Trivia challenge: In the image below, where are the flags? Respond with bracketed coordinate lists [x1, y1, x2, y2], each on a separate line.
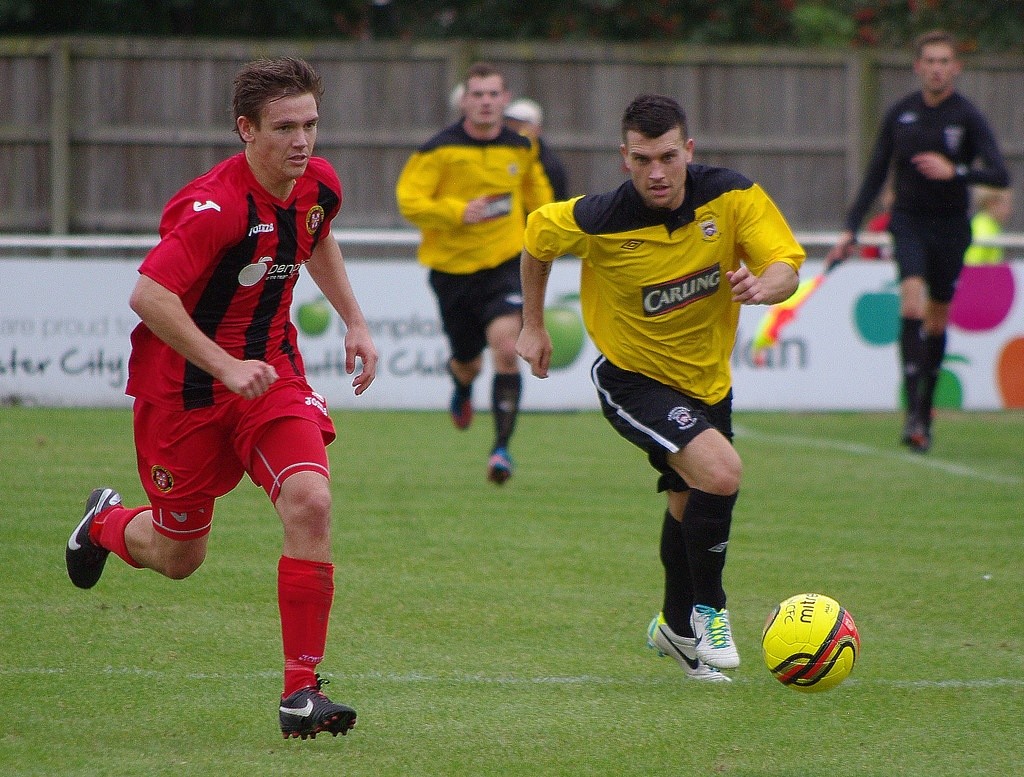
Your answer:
[753, 272, 821, 349]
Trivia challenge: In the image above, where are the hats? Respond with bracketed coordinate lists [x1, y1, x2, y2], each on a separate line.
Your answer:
[504, 99, 543, 123]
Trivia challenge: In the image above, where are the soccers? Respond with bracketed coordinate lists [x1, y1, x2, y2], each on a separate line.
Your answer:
[761, 592, 862, 692]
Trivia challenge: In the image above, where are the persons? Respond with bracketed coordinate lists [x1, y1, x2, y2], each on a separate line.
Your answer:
[63, 55, 360, 739]
[823, 32, 1012, 452]
[396, 63, 556, 486]
[861, 176, 896, 259]
[964, 184, 1012, 266]
[500, 99, 568, 219]
[515, 92, 806, 685]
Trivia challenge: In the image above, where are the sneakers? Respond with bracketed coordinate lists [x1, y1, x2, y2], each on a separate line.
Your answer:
[901, 413, 925, 443]
[647, 611, 733, 682]
[451, 385, 472, 430]
[488, 447, 512, 484]
[689, 606, 741, 670]
[915, 423, 933, 452]
[66, 488, 122, 589]
[278, 687, 357, 740]
